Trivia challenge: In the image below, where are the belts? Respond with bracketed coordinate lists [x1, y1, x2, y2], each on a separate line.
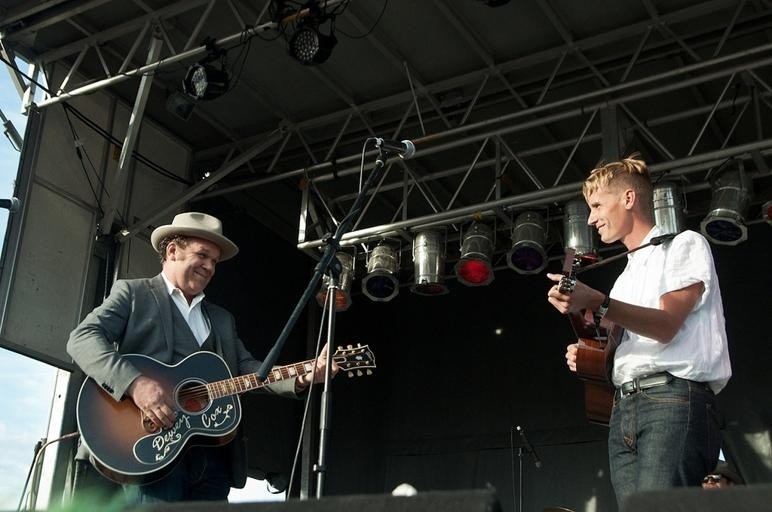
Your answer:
[613, 371, 714, 398]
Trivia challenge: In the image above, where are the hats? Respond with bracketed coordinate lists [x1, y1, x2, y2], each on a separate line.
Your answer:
[150, 212, 240, 264]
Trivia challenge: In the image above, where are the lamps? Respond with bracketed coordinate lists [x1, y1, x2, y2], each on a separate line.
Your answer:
[317, 169, 752, 312]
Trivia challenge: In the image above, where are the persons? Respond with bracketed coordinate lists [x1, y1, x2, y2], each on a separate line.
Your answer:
[701, 457, 744, 490]
[65, 209, 342, 507]
[545, 153, 734, 511]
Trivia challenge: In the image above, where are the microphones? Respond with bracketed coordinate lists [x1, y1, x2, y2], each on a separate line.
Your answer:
[61, 432, 80, 440]
[514, 426, 543, 469]
[374, 138, 416, 159]
[0, 196, 23, 212]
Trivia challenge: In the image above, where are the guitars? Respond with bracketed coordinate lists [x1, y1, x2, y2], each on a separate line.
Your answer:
[76, 342, 377, 485]
[559, 247, 624, 427]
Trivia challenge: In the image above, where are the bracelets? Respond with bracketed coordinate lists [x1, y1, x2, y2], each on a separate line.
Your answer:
[592, 296, 611, 329]
